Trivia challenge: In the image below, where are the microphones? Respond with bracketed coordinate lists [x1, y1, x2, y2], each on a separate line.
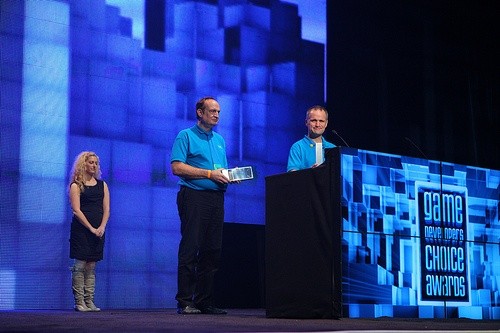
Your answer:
[405, 138, 427, 159]
[332, 130, 350, 148]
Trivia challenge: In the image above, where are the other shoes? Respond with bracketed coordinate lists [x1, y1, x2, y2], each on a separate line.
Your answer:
[202, 305, 228, 314]
[74, 302, 100, 311]
[176, 303, 201, 314]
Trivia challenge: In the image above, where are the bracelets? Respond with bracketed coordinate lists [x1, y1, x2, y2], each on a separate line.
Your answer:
[207, 170, 211, 179]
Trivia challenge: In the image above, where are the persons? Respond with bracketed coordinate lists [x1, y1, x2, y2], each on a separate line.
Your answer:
[287, 105, 337, 172]
[170, 97, 241, 315]
[69, 151, 110, 311]
